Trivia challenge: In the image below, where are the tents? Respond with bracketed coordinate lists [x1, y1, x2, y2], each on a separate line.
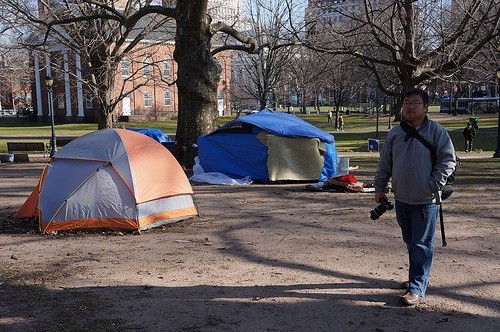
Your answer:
[197, 109, 337, 184]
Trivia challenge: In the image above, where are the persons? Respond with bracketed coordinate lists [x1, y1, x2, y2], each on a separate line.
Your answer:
[463, 122, 476, 153]
[337, 114, 344, 131]
[8, 128, 200, 235]
[327, 110, 332, 123]
[373, 88, 457, 304]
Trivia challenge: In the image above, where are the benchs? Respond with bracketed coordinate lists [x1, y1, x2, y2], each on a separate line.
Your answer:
[46, 136, 81, 151]
[7, 142, 48, 158]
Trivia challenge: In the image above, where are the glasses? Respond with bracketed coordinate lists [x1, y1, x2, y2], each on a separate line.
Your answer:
[402, 101, 422, 106]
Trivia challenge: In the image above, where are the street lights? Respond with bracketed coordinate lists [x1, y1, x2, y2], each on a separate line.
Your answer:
[43, 73, 58, 157]
[493, 68, 500, 158]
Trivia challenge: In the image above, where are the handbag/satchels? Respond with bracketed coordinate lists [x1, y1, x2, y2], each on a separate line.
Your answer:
[432, 148, 458, 185]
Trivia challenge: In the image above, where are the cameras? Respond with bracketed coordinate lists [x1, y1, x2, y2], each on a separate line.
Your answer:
[370, 197, 393, 220]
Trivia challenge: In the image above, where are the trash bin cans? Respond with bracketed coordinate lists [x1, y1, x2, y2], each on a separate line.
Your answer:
[469, 118, 479, 128]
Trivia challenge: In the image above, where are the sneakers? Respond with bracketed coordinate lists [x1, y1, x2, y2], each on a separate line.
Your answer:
[402, 281, 410, 289]
[400, 290, 421, 305]
